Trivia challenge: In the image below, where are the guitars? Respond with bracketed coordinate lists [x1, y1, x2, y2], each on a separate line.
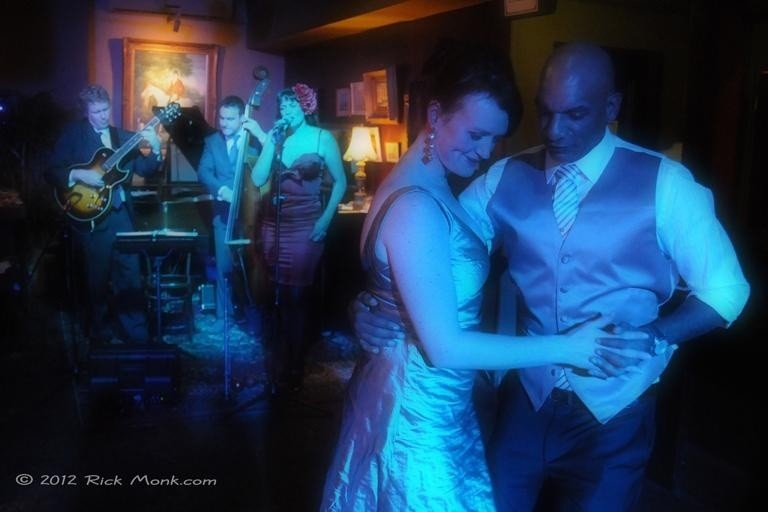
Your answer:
[54, 102, 181, 228]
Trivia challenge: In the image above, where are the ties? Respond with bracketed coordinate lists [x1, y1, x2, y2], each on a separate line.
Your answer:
[229, 135, 240, 165]
[552, 163, 581, 391]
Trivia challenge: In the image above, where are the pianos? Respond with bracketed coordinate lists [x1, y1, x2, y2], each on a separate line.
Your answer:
[124, 104, 216, 239]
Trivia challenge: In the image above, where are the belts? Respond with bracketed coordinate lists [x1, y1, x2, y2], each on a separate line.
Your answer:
[548, 389, 577, 405]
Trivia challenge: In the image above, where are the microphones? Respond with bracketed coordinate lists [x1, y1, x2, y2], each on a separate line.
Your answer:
[272, 115, 289, 136]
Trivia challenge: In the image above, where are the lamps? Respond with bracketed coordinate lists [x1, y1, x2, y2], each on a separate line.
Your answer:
[342, 125, 379, 210]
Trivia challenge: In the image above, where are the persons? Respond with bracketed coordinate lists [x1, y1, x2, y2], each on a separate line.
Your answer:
[344, 37, 750, 512]
[44, 83, 162, 345]
[316, 63, 654, 511]
[195, 95, 273, 336]
[250, 86, 347, 391]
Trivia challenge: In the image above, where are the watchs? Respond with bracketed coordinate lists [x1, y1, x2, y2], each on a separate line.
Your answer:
[642, 323, 668, 357]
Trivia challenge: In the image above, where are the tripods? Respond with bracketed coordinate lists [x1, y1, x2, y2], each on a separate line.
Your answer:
[216, 146, 334, 427]
[41, 225, 90, 421]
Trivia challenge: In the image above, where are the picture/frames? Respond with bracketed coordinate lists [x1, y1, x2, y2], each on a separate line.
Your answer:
[336, 65, 400, 126]
[503, 0, 538, 17]
[122, 34, 220, 140]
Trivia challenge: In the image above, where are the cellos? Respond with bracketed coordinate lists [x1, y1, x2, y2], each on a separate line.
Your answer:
[229, 69, 276, 313]
